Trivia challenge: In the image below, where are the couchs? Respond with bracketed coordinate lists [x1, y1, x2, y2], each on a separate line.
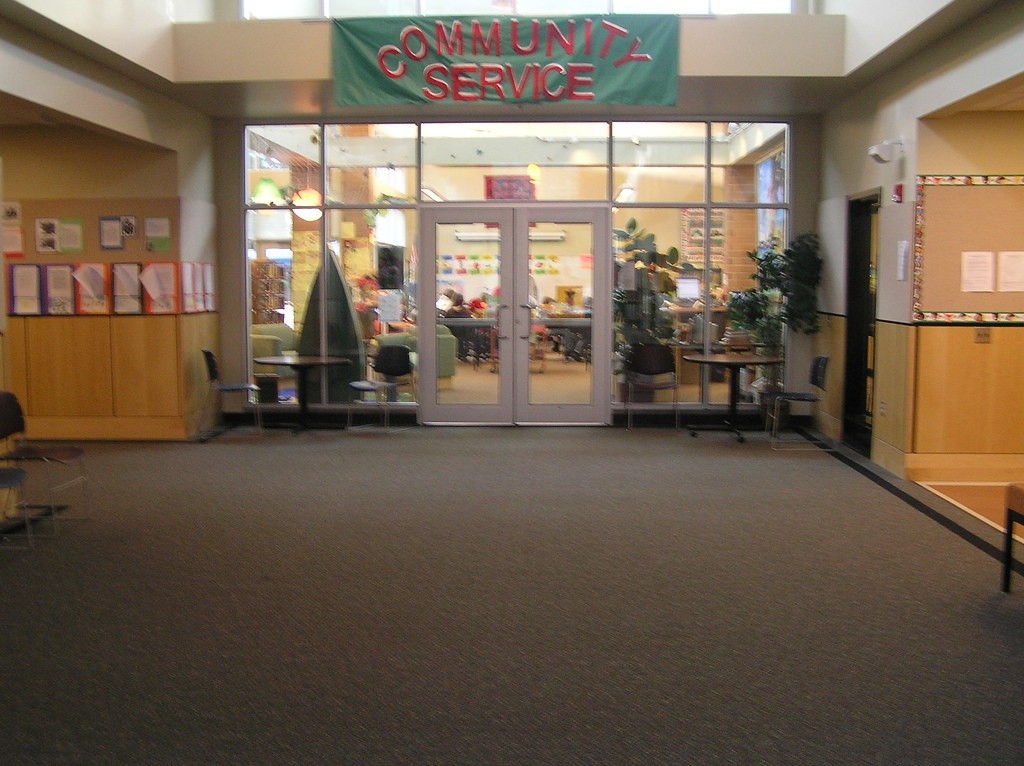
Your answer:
[247, 322, 457, 379]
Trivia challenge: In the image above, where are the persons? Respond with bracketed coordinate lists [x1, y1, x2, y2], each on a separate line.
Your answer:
[436, 289, 499, 355]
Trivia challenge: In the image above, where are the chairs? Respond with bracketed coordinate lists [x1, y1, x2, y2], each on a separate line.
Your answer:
[347, 344, 424, 435]
[626, 344, 680, 433]
[0, 391, 94, 551]
[762, 355, 840, 452]
[193, 346, 267, 444]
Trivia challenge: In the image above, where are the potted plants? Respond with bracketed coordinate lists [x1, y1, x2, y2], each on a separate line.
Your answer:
[609, 216, 696, 404]
[727, 229, 825, 407]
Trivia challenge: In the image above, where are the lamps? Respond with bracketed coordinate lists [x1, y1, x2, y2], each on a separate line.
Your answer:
[612, 185, 638, 204]
[249, 176, 325, 221]
[455, 230, 568, 242]
[370, 161, 407, 248]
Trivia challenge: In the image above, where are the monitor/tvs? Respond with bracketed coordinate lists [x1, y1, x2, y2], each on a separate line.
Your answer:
[675, 276, 701, 302]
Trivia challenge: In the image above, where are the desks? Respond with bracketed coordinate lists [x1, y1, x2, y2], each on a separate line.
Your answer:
[252, 356, 353, 435]
[682, 351, 785, 445]
